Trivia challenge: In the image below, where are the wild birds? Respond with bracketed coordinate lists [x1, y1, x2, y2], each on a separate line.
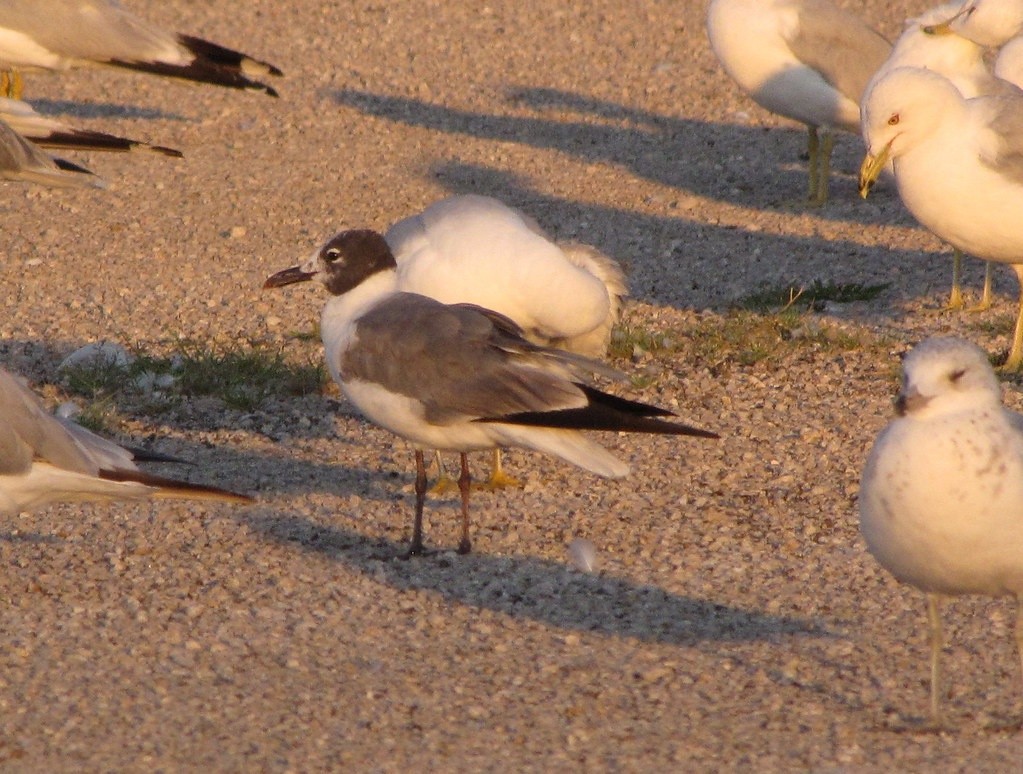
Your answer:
[0, 345, 257, 540]
[0, 1, 284, 191]
[859, 0, 1023, 379]
[259, 188, 722, 568]
[858, 336, 1023, 736]
[706, 1, 896, 211]
